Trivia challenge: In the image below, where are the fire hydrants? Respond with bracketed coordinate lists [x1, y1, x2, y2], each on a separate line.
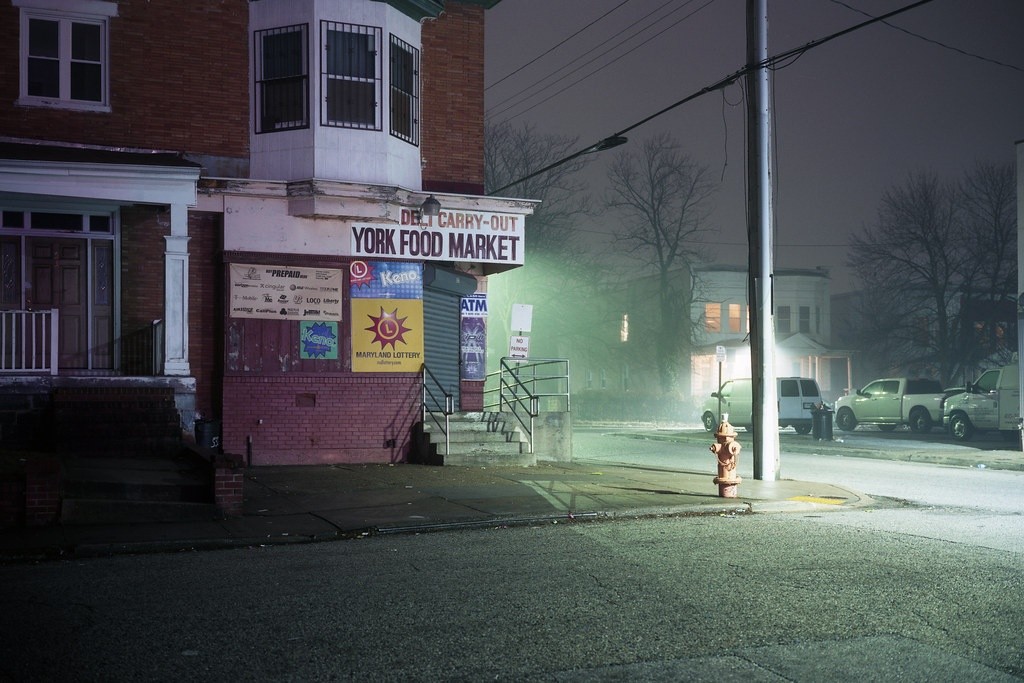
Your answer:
[707, 413, 743, 497]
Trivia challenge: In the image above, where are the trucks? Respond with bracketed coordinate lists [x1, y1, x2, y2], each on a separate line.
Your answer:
[944, 364, 1023, 441]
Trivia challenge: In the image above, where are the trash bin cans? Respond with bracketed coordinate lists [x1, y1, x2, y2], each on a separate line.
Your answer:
[810, 401, 835, 441]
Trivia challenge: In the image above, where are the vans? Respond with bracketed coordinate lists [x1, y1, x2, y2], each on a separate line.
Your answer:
[701, 376, 823, 435]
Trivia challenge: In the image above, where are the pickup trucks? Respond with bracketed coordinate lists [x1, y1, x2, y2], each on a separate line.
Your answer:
[833, 378, 959, 432]
